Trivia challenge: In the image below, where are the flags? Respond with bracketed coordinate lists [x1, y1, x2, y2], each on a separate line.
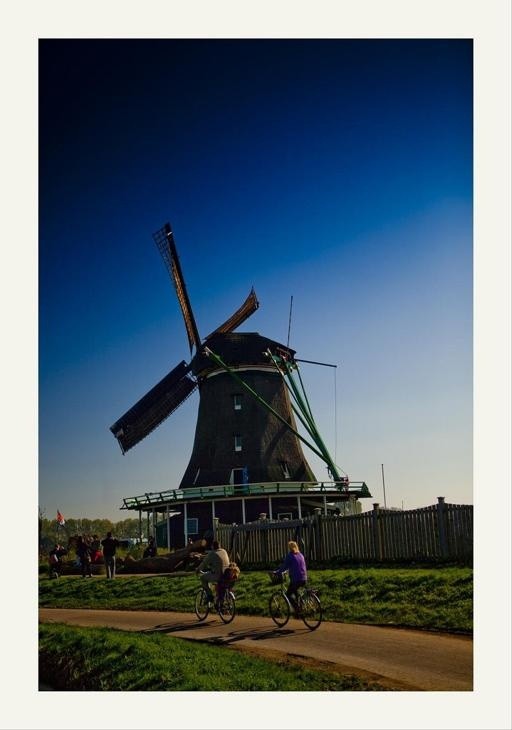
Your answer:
[57, 511, 65, 525]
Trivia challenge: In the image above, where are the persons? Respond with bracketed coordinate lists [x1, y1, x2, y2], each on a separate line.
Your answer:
[143, 536, 157, 559]
[195, 541, 229, 602]
[76, 532, 118, 579]
[214, 563, 240, 601]
[273, 541, 307, 619]
[49, 545, 68, 579]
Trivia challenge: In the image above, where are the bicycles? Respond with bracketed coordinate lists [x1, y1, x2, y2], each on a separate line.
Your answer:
[266, 570, 324, 631]
[194, 568, 237, 624]
